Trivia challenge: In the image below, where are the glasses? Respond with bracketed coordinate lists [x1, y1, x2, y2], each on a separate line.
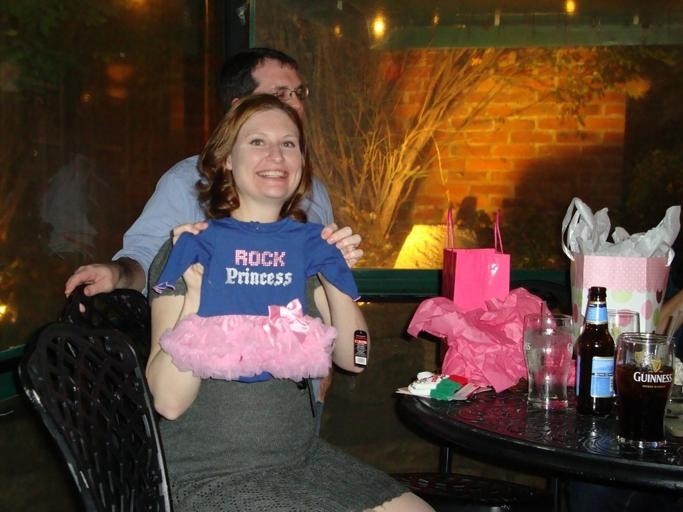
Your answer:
[268, 82, 311, 102]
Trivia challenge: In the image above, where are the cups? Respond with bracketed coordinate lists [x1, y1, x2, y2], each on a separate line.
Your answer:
[614, 332, 675, 447]
[522, 313, 574, 408]
[574, 287, 617, 419]
[605, 308, 640, 347]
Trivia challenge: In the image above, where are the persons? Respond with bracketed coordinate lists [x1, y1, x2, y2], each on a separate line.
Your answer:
[63, 46, 366, 315]
[141, 90, 439, 512]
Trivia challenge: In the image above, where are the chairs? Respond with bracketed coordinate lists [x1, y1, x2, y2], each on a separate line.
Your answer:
[59, 284, 161, 426]
[14, 323, 170, 511]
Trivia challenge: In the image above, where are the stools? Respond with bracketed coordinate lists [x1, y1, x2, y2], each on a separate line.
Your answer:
[387, 472, 549, 512]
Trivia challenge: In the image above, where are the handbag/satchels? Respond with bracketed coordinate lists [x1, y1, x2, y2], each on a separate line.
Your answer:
[441, 247, 512, 390]
[569, 249, 676, 359]
